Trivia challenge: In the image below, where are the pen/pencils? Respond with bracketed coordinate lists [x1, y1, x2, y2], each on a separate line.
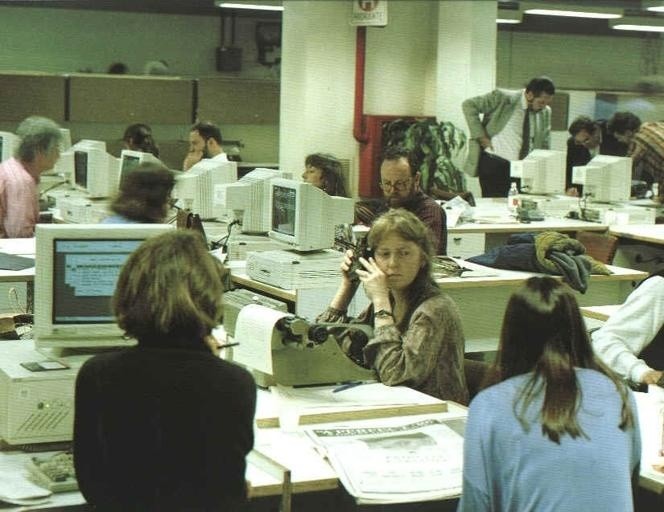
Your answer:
[332, 381, 364, 395]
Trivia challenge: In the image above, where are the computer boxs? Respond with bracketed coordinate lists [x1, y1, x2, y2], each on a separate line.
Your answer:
[507, 195, 578, 218]
[577, 202, 657, 229]
[44, 190, 111, 225]
[247, 249, 348, 291]
[0, 339, 100, 446]
[206, 229, 271, 261]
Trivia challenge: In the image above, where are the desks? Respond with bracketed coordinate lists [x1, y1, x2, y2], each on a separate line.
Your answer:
[576, 303, 628, 322]
[350, 197, 609, 258]
[628, 384, 664, 492]
[0, 325, 469, 512]
[518, 193, 663, 245]
[229, 253, 649, 341]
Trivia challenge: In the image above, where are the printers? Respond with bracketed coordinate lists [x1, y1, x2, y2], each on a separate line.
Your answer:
[222, 287, 383, 389]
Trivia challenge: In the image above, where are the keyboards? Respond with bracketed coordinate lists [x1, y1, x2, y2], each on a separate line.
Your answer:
[26, 453, 79, 494]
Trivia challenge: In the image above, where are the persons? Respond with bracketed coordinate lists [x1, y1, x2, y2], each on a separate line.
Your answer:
[101, 162, 178, 224]
[184, 121, 228, 173]
[590, 268, 664, 392]
[607, 112, 664, 193]
[356, 149, 447, 256]
[315, 208, 470, 407]
[301, 153, 346, 197]
[566, 115, 627, 199]
[0, 115, 62, 238]
[124, 123, 159, 158]
[73, 230, 257, 512]
[457, 276, 641, 512]
[462, 76, 556, 198]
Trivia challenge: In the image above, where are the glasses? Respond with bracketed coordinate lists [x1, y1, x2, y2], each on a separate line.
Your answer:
[379, 178, 410, 192]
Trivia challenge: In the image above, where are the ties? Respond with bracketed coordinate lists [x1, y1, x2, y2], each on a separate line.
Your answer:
[518, 110, 530, 160]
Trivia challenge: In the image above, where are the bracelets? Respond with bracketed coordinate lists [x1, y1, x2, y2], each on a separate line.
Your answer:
[374, 309, 393, 318]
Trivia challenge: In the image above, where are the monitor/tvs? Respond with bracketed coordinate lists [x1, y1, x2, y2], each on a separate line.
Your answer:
[0, 131, 21, 162]
[212, 166, 294, 236]
[266, 176, 356, 253]
[572, 155, 634, 204]
[507, 147, 567, 196]
[33, 223, 176, 348]
[54, 138, 107, 179]
[70, 145, 118, 199]
[172, 157, 237, 222]
[113, 149, 165, 198]
